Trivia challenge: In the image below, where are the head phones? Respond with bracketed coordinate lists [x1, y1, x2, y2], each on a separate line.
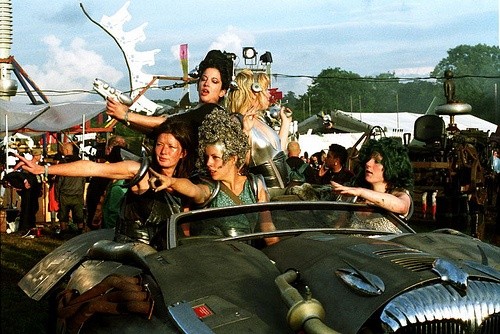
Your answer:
[251, 73, 262, 92]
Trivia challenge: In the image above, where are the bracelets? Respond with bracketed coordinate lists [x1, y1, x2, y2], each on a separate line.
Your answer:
[44, 165, 48, 176]
[124, 111, 129, 122]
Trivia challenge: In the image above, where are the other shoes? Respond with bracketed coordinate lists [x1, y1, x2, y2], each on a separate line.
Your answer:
[22, 230, 34, 239]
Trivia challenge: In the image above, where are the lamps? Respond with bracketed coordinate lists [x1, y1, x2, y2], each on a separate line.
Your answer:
[243, 47, 272, 68]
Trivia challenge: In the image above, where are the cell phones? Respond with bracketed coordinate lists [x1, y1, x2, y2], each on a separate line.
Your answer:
[325, 164, 330, 169]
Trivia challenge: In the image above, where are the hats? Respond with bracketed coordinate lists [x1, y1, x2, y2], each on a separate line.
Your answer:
[32, 149, 42, 156]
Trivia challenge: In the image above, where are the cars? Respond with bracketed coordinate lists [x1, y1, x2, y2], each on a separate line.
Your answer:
[17, 198, 500, 334]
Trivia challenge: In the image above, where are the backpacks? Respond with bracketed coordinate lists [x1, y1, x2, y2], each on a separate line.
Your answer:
[285, 161, 309, 184]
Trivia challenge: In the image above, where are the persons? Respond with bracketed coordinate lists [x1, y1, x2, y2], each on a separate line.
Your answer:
[105, 49, 234, 173]
[148, 108, 279, 245]
[227, 70, 293, 189]
[0, 132, 127, 236]
[286, 122, 500, 237]
[13, 120, 190, 251]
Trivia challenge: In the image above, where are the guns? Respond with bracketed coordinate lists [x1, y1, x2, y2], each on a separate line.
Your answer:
[270, 104, 293, 119]
[92, 77, 134, 107]
